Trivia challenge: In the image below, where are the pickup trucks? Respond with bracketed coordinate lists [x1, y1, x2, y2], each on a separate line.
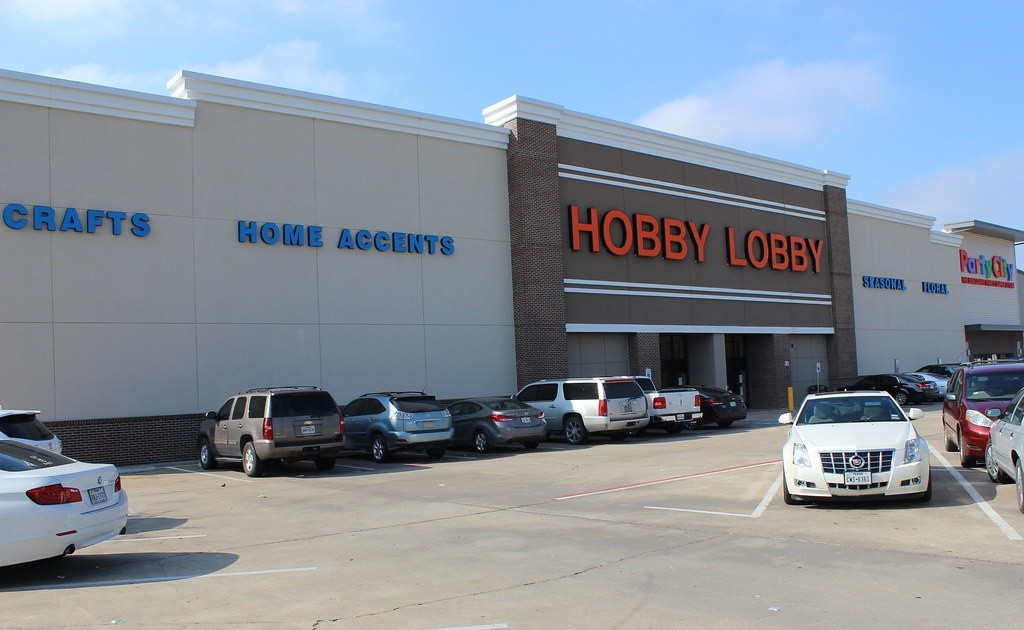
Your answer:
[594, 375, 704, 435]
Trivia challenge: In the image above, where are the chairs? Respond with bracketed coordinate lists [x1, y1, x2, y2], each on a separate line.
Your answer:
[808, 401, 836, 423]
[970, 378, 995, 394]
[861, 405, 889, 419]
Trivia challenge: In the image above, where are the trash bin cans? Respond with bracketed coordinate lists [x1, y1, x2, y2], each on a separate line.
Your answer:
[808, 384, 830, 395]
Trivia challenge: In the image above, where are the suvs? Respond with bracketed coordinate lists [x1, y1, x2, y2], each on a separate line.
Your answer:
[334, 391, 456, 464]
[942, 358, 1024, 468]
[197, 385, 347, 477]
[510, 376, 650, 446]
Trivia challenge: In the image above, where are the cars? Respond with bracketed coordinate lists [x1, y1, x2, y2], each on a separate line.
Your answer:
[836, 373, 940, 405]
[778, 390, 932, 504]
[0, 408, 64, 459]
[661, 384, 747, 430]
[913, 362, 979, 388]
[0, 439, 130, 570]
[901, 371, 952, 402]
[443, 396, 549, 453]
[984, 387, 1024, 513]
